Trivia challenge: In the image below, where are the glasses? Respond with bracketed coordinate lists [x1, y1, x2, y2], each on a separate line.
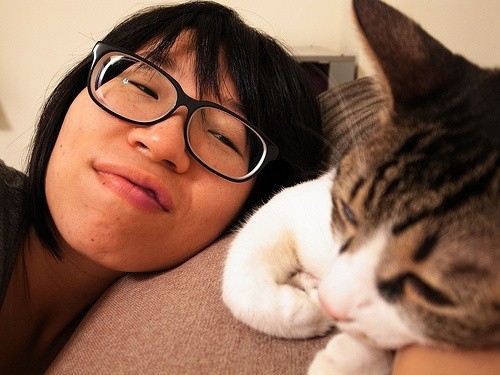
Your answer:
[87, 40, 278, 182]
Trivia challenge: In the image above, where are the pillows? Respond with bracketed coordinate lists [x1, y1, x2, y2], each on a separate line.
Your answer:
[312, 76, 388, 169]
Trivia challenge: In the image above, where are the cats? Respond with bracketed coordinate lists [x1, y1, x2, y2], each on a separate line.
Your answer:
[219, 0, 500, 375]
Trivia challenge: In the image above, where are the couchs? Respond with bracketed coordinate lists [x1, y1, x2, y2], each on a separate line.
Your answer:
[42, 212, 500, 375]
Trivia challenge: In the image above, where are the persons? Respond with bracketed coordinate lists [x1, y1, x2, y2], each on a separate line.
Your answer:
[1, 1, 326, 375]
